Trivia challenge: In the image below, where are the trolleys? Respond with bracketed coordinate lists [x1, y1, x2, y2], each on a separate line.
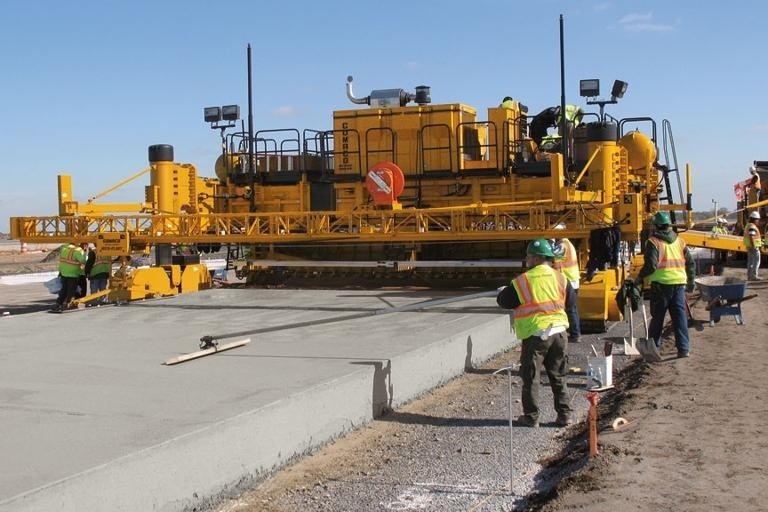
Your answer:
[694, 275, 758, 327]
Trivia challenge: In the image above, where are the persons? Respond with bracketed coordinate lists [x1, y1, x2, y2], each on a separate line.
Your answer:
[495, 240, 573, 429]
[722, 217, 730, 268]
[710, 218, 724, 270]
[77, 242, 95, 299]
[742, 211, 764, 282]
[498, 95, 528, 115]
[635, 211, 697, 356]
[51, 242, 89, 313]
[88, 246, 113, 305]
[551, 223, 582, 343]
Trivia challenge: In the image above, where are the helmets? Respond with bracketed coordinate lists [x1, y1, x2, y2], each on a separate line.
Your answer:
[749, 211, 761, 220]
[652, 211, 671, 226]
[717, 218, 727, 224]
[527, 239, 555, 258]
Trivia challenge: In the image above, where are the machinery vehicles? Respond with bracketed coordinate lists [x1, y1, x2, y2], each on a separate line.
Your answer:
[10, 13, 768, 332]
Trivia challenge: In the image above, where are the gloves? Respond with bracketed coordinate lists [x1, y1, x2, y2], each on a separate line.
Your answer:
[633, 276, 644, 286]
[684, 285, 695, 292]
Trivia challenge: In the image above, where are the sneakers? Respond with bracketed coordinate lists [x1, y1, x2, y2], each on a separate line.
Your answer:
[567, 335, 582, 343]
[556, 416, 572, 426]
[518, 414, 540, 428]
[749, 276, 763, 281]
[678, 351, 690, 358]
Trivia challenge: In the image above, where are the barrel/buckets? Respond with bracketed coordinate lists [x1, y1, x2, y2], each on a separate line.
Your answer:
[585, 351, 613, 387]
[44, 275, 62, 294]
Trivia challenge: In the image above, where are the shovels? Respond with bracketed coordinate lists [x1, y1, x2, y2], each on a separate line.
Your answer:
[624, 297, 662, 362]
